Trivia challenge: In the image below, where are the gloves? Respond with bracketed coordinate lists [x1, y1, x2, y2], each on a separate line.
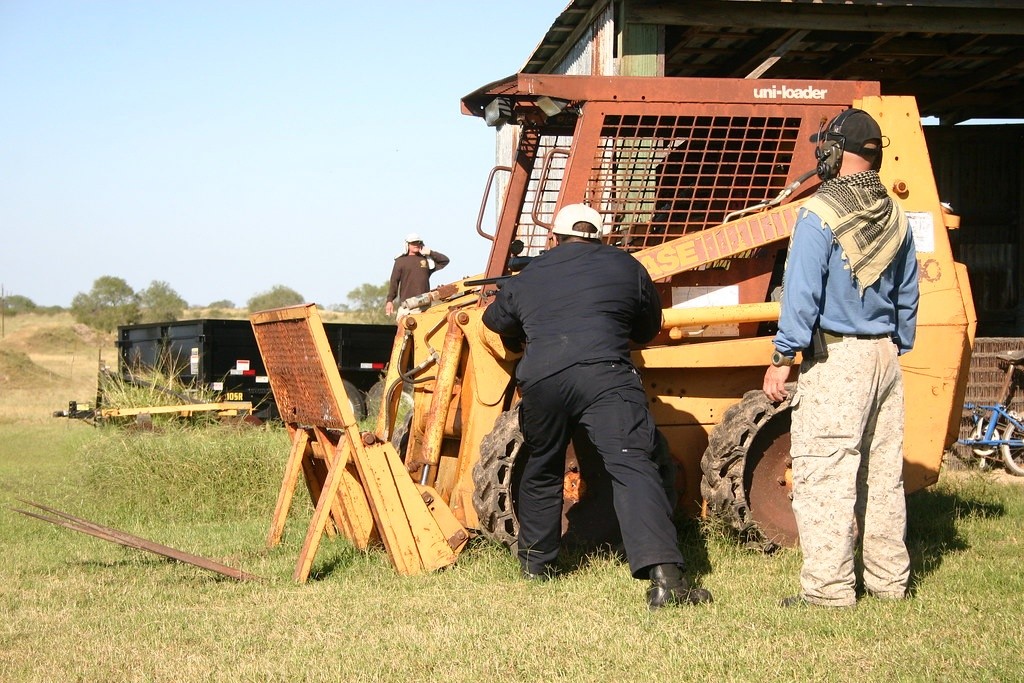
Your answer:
[419, 246, 431, 255]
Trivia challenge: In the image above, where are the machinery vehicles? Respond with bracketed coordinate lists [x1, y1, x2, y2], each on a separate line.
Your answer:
[247, 72, 975, 566]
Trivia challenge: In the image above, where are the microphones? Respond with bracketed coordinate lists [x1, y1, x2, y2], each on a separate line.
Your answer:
[815, 115, 828, 159]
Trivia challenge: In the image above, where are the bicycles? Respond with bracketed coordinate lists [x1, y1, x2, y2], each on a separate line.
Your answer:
[956, 350, 1024, 477]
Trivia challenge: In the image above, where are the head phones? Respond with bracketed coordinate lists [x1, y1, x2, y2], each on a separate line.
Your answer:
[814, 108, 884, 180]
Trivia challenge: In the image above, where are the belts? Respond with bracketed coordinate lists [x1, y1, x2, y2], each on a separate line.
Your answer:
[822, 330, 888, 345]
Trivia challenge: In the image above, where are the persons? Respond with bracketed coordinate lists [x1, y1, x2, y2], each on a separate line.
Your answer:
[385, 232, 451, 321]
[762, 107, 922, 612]
[481, 203, 715, 609]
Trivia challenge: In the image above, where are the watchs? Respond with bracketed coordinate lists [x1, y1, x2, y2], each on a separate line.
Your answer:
[770, 351, 792, 367]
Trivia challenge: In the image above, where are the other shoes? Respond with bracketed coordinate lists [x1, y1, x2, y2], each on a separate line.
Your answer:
[781, 594, 808, 607]
[647, 576, 715, 612]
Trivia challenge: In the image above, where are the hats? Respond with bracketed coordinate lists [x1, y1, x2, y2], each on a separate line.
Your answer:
[394, 233, 436, 270]
[808, 107, 882, 157]
[552, 202, 604, 240]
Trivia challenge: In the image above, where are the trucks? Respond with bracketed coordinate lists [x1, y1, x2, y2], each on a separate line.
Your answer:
[113, 318, 414, 422]
